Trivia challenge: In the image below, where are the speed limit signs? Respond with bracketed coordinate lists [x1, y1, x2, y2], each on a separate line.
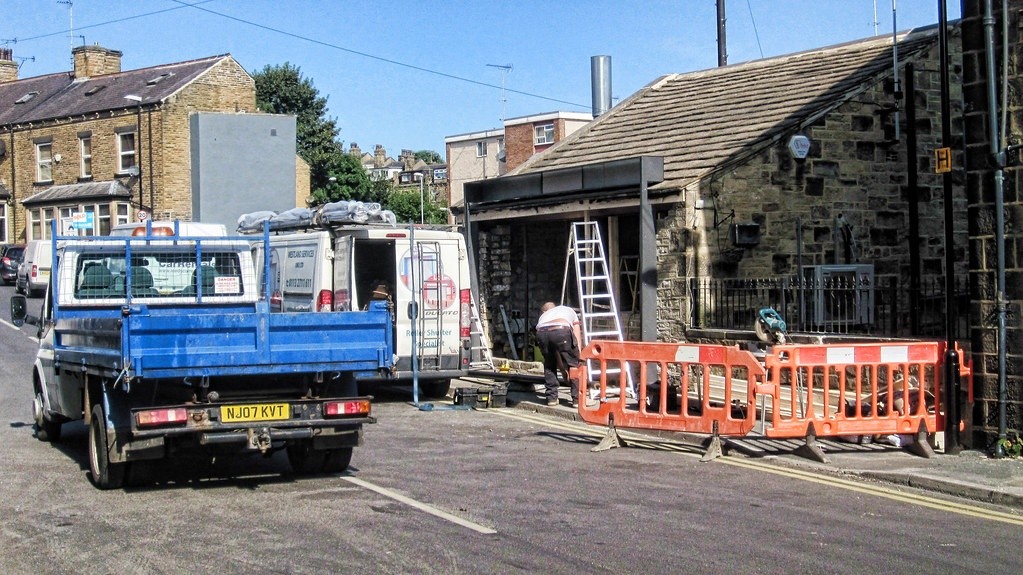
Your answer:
[137, 210, 147, 219]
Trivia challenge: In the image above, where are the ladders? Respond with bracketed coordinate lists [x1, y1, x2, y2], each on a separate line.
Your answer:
[560, 220, 637, 402]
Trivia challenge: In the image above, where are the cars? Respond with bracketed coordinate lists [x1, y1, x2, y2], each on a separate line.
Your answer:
[0, 228, 62, 291]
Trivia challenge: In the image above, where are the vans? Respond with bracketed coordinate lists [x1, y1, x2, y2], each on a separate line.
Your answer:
[234, 201, 480, 401]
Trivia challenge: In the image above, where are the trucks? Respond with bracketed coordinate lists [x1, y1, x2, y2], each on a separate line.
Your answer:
[30, 219, 397, 487]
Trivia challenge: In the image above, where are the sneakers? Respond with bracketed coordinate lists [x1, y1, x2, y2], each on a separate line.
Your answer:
[572, 398, 596, 407]
[548, 399, 559, 406]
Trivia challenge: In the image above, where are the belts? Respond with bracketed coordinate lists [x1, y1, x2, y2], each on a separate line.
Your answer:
[537, 326, 569, 331]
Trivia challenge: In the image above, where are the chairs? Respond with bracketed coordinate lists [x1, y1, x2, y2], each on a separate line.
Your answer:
[73, 265, 116, 299]
[116, 267, 161, 297]
[184, 265, 219, 294]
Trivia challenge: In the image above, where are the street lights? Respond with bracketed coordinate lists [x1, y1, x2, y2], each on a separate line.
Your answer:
[414, 171, 424, 224]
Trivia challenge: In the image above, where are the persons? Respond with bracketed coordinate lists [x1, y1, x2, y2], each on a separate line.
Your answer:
[536, 301, 585, 409]
[82, 261, 101, 278]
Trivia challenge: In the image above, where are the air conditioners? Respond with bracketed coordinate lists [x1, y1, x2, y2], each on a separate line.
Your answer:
[802, 264, 875, 327]
